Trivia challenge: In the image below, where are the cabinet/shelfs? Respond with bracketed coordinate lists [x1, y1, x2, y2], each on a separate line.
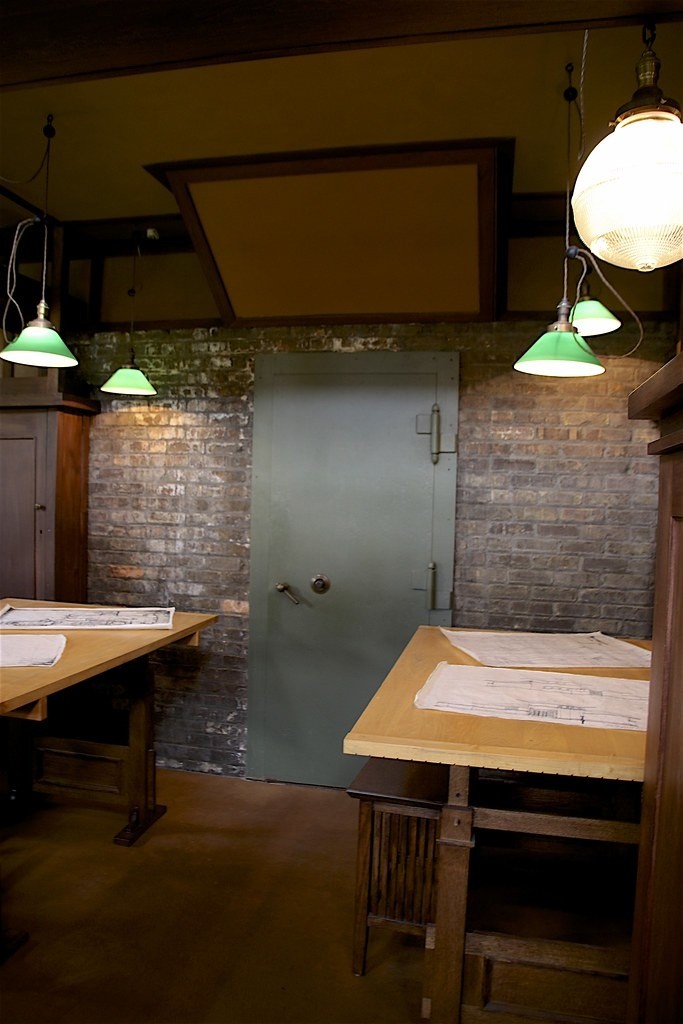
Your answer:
[0, 396, 102, 603]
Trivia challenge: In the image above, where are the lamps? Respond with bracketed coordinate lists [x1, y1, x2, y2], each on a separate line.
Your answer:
[0, 115, 82, 367]
[515, 62, 609, 376]
[561, 21, 683, 274]
[569, 26, 624, 337]
[101, 244, 159, 397]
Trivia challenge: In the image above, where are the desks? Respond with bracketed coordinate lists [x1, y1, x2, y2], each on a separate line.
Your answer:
[0, 599, 224, 848]
[351, 625, 655, 1024]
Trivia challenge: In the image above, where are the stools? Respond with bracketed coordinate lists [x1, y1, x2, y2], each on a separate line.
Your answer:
[351, 754, 480, 973]
